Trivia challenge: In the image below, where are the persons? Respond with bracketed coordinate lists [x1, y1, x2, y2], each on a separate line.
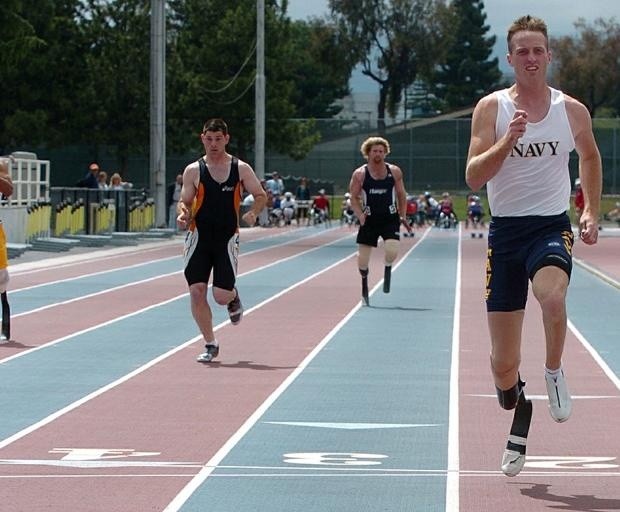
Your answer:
[348, 136, 413, 308]
[76, 163, 133, 232]
[393, 189, 458, 237]
[466, 188, 491, 239]
[0, 157, 19, 345]
[177, 115, 271, 364]
[573, 177, 602, 237]
[166, 174, 183, 225]
[342, 188, 364, 226]
[465, 13, 605, 477]
[603, 199, 620, 223]
[239, 171, 331, 228]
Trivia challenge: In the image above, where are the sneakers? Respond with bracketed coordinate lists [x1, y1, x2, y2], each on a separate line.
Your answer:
[227, 287, 243, 325]
[546, 369, 572, 423]
[196, 343, 219, 362]
